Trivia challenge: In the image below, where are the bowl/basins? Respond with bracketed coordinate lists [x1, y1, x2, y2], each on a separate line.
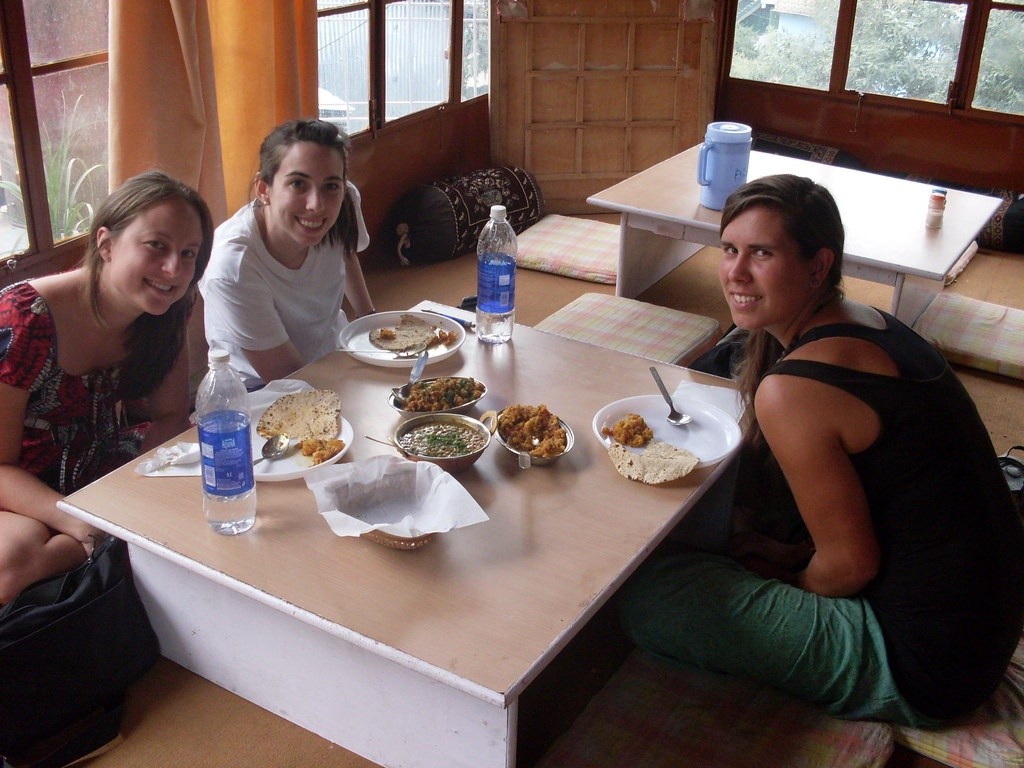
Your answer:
[388, 376, 488, 420]
[491, 408, 576, 466]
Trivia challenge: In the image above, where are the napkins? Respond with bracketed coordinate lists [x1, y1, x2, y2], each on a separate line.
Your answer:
[134, 441, 201, 477]
[247, 379, 315, 409]
[671, 380, 752, 424]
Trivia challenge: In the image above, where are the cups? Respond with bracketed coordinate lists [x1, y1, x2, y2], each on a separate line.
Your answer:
[698, 121, 753, 211]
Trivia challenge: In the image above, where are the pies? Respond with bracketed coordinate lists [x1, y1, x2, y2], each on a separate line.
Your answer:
[256, 389, 342, 441]
[607, 441, 700, 485]
[369, 314, 436, 350]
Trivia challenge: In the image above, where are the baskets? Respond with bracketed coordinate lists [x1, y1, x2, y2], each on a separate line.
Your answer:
[327, 466, 439, 551]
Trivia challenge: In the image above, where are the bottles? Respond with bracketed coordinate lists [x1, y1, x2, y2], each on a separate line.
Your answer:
[195, 349, 256, 536]
[476, 206, 517, 343]
[926, 187, 947, 232]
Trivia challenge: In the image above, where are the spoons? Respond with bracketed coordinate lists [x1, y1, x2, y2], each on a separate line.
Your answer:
[253, 433, 290, 470]
[334, 343, 428, 357]
[649, 366, 692, 426]
[391, 351, 428, 404]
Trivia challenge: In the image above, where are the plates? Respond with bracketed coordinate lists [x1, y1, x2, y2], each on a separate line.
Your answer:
[247, 409, 354, 482]
[339, 311, 466, 369]
[593, 395, 743, 470]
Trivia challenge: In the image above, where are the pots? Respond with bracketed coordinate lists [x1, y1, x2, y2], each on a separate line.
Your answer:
[388, 411, 499, 476]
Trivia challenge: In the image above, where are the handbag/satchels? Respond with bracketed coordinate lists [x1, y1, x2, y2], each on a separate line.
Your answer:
[0, 535, 162, 768]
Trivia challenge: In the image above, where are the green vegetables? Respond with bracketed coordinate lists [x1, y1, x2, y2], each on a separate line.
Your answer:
[425, 432, 468, 455]
[408, 376, 477, 408]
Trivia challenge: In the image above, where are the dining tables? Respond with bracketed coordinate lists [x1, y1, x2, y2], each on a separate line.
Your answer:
[54, 298, 757, 768]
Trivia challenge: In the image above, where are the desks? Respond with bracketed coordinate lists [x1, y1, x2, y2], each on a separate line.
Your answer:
[586, 142, 1003, 330]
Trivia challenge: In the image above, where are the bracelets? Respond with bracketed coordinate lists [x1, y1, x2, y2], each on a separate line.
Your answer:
[355, 309, 376, 319]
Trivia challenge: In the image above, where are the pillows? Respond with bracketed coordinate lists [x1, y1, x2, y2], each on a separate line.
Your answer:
[752, 131, 864, 172]
[396, 164, 543, 267]
[860, 168, 1024, 256]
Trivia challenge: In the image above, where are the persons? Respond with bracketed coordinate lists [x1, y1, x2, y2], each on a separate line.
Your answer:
[196, 120, 374, 392]
[614, 174, 1024, 732]
[0, 171, 212, 606]
